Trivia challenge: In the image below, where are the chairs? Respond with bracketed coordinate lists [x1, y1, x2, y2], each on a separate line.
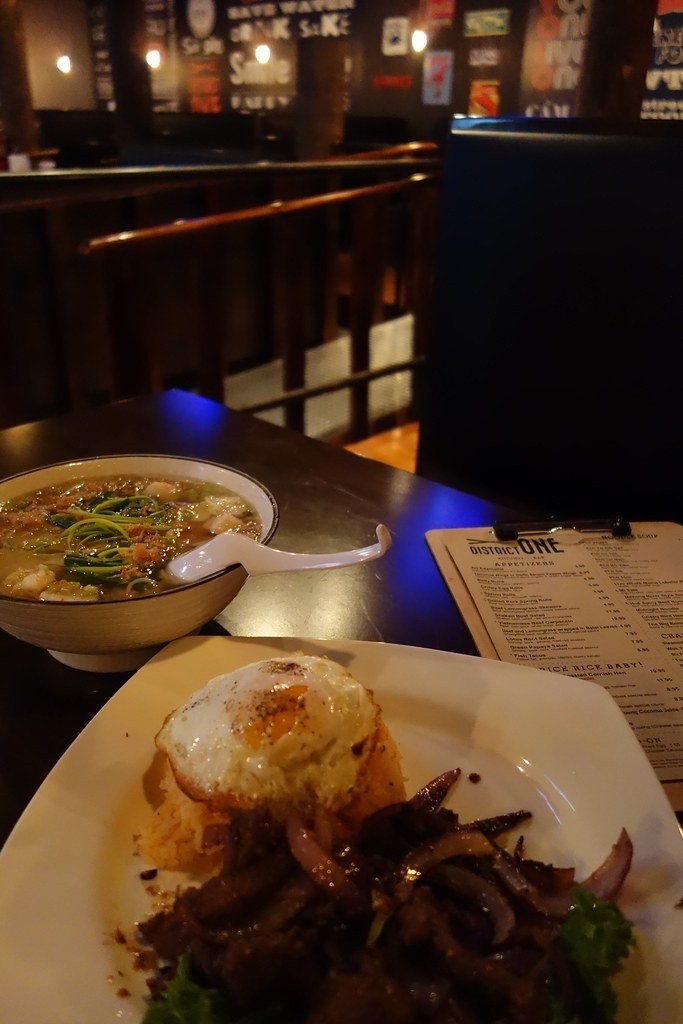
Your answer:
[414, 117, 683, 524]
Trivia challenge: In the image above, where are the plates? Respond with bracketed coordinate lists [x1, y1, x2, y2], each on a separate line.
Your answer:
[0, 635, 683, 1024]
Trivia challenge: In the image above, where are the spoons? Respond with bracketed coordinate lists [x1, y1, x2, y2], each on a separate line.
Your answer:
[167, 524, 399, 583]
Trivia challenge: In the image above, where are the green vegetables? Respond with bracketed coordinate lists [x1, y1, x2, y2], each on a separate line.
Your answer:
[142, 882, 638, 1024]
[24, 489, 192, 594]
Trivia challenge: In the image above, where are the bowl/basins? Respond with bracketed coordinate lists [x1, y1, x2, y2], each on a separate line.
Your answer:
[1, 454, 280, 673]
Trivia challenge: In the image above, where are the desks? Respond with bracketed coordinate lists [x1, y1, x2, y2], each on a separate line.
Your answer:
[0, 387, 683, 850]
